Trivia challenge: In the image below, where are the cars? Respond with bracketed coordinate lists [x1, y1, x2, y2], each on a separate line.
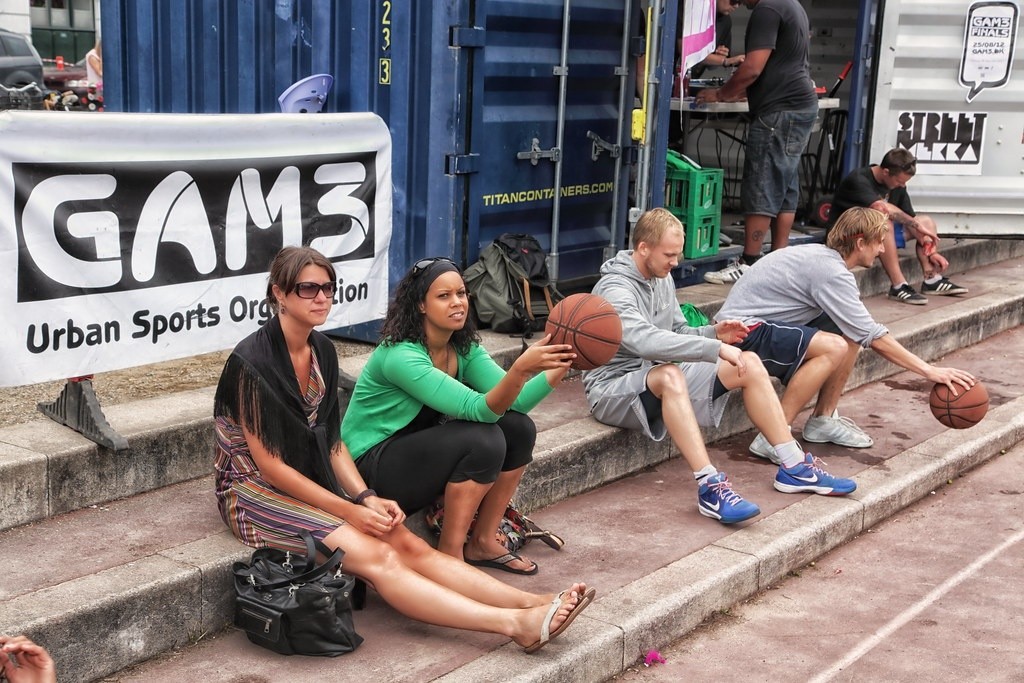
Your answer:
[44, 56, 88, 105]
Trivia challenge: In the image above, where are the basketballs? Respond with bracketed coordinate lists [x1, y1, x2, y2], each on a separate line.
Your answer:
[929, 376, 990, 430]
[545, 292, 623, 370]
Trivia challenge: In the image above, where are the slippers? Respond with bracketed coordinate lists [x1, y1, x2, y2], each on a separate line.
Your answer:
[525, 586, 596, 652]
[466, 547, 538, 575]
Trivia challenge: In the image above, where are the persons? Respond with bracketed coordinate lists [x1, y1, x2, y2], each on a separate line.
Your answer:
[581, 208, 856, 523]
[213, 246, 595, 655]
[340, 260, 577, 575]
[87, 43, 102, 85]
[714, 208, 974, 465]
[669, 0, 746, 151]
[0, 635, 56, 683]
[826, 149, 969, 305]
[697, 0, 818, 285]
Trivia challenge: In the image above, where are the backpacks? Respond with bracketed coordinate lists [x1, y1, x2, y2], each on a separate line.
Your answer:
[462, 232, 565, 337]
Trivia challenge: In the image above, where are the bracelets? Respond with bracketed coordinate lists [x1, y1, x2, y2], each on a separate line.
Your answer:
[723, 58, 727, 68]
[354, 489, 377, 505]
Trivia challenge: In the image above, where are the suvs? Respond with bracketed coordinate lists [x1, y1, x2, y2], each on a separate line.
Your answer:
[0, 28, 44, 110]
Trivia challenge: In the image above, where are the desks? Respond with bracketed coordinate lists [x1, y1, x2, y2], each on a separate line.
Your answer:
[671, 96, 840, 227]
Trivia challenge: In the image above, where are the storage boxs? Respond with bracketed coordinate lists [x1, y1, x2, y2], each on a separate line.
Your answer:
[664, 166, 724, 260]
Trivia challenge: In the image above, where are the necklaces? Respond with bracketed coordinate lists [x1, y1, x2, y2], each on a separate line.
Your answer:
[447, 344, 448, 374]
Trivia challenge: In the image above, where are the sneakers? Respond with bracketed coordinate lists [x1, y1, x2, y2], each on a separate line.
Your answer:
[774, 452, 857, 495]
[889, 284, 928, 304]
[922, 277, 968, 295]
[697, 472, 760, 523]
[749, 426, 805, 466]
[802, 408, 874, 447]
[703, 252, 764, 284]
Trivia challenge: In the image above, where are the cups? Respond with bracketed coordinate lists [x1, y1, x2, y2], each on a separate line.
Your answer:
[56, 56, 64, 70]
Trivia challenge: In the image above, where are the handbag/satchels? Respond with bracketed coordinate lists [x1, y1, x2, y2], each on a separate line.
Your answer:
[425, 499, 565, 553]
[231, 530, 364, 657]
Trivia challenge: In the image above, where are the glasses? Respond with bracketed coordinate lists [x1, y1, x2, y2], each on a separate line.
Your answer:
[729, 0, 742, 6]
[285, 281, 336, 299]
[411, 257, 456, 280]
